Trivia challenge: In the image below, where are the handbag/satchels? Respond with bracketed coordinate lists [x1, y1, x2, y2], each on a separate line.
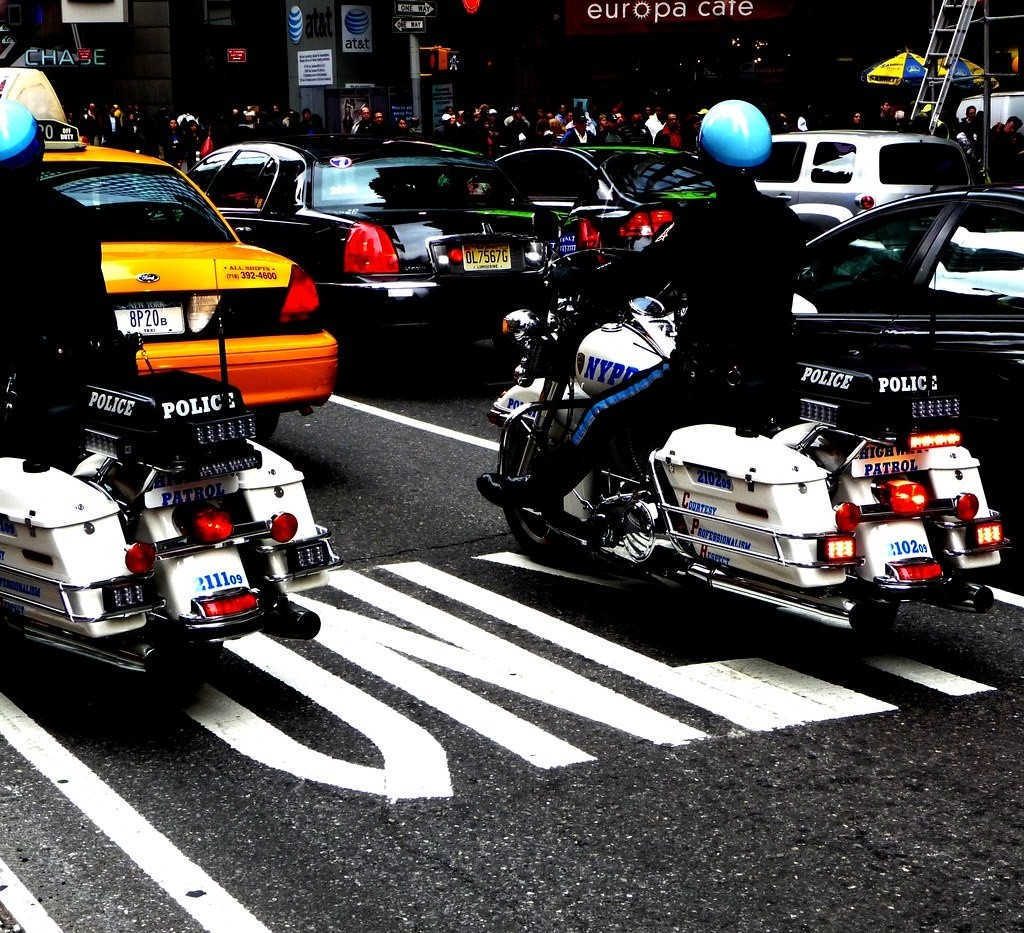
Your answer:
[200, 127, 213, 158]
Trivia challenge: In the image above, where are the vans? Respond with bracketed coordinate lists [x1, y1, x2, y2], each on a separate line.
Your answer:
[748, 129, 980, 241]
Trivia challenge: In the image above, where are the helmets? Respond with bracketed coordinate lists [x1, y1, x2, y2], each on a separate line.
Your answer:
[700, 100, 773, 177]
[0, 84, 44, 171]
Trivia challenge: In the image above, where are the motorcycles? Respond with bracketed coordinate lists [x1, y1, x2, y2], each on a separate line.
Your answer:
[483, 224, 1005, 636]
[0, 341, 344, 711]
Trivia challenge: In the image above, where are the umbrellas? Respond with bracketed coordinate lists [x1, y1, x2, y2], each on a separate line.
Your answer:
[860, 51, 951, 86]
[934, 57, 999, 89]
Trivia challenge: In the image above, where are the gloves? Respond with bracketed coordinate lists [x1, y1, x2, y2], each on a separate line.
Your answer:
[547, 264, 585, 294]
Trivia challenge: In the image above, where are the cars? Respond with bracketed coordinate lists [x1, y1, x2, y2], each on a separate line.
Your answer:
[804, 184, 1024, 512]
[175, 131, 580, 359]
[0, 65, 342, 453]
[462, 145, 727, 249]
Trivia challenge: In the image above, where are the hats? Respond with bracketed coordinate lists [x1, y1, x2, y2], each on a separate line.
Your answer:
[506, 103, 700, 131]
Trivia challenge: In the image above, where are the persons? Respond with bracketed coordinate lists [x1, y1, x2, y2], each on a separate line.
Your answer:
[478, 99, 805, 507]
[0, 105, 122, 461]
[65, 103, 1024, 186]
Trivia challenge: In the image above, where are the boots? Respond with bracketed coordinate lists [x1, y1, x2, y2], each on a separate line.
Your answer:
[479, 437, 593, 521]
